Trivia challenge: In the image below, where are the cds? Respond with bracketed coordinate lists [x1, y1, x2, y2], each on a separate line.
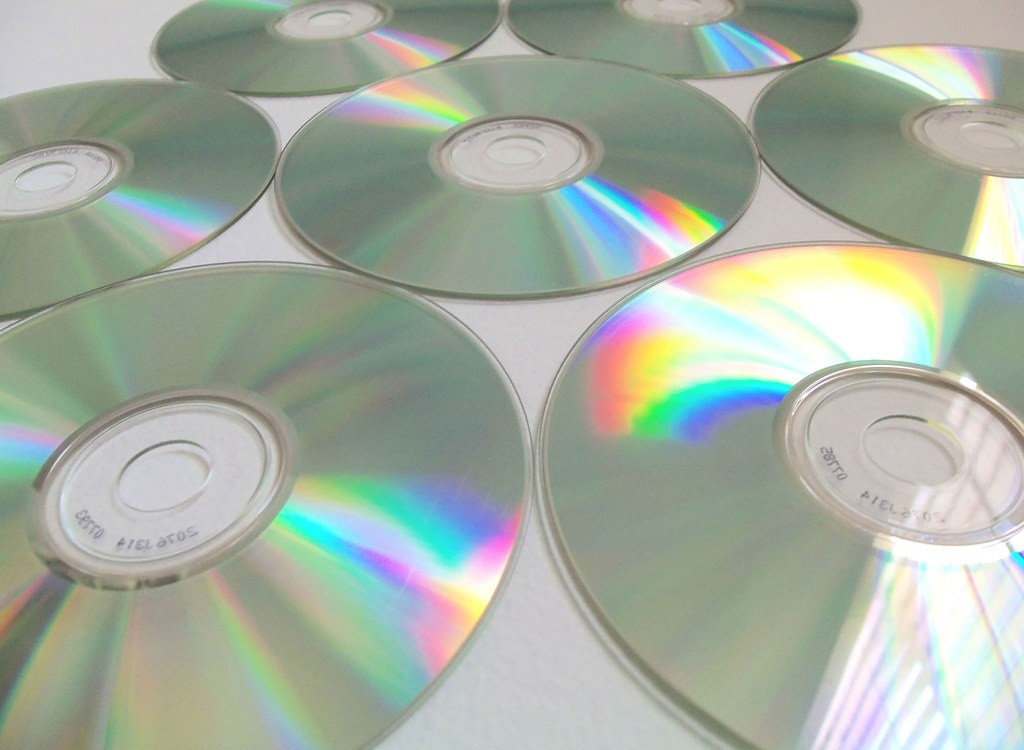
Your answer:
[1, 0, 1023, 750]
